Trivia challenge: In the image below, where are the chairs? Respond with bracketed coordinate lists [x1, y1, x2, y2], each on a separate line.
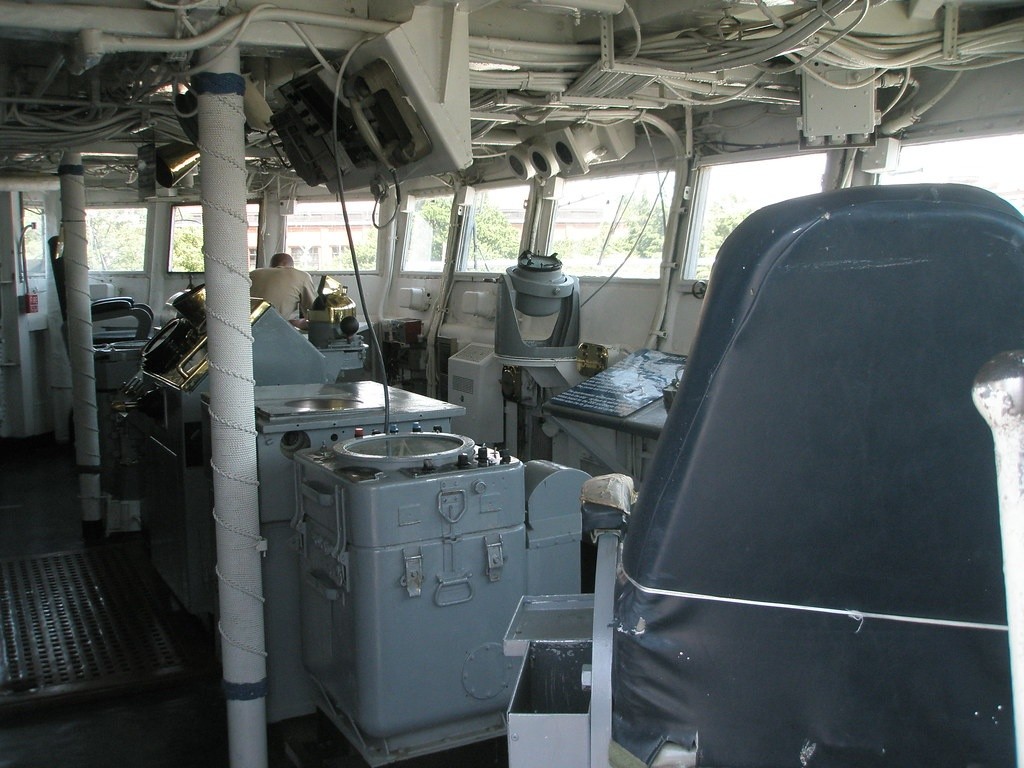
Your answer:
[578, 184, 1024, 768]
[48, 234, 154, 359]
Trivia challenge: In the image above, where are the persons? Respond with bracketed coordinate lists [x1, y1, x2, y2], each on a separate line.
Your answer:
[250, 252, 317, 330]
